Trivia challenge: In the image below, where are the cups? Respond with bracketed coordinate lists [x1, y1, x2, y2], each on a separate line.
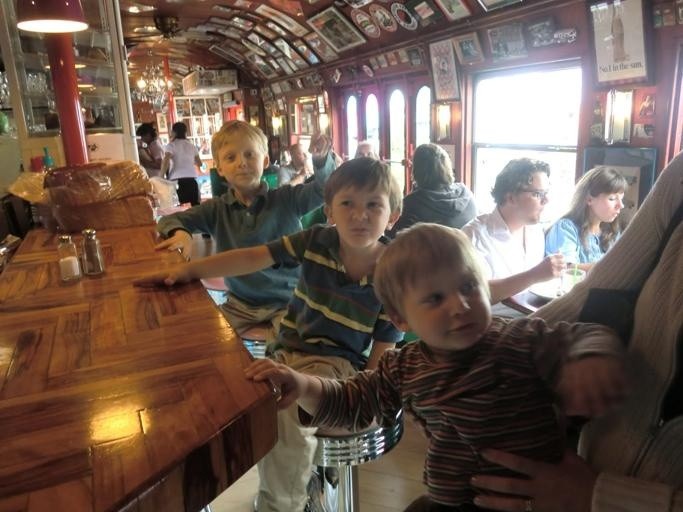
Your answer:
[562, 268, 587, 293]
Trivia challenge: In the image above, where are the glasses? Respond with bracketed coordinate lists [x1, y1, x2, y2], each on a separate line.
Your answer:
[523, 189, 549, 200]
[519, 188, 550, 200]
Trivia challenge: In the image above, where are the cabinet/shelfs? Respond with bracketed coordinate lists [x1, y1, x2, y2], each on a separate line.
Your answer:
[0, 0, 140, 172]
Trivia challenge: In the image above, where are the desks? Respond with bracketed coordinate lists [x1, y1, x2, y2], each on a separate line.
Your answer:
[499, 288, 553, 316]
[0, 224, 282, 512]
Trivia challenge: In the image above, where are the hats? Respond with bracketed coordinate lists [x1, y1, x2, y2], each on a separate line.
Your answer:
[173, 122, 188, 134]
[136, 122, 153, 136]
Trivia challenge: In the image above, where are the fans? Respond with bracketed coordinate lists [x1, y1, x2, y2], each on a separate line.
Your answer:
[124, 15, 212, 50]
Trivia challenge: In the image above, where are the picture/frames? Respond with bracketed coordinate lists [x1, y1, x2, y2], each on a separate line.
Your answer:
[244, 11, 266, 23]
[452, 28, 488, 70]
[425, 35, 463, 104]
[290, 38, 319, 67]
[252, 22, 278, 42]
[586, 0, 655, 88]
[582, 146, 658, 229]
[273, 38, 310, 72]
[252, 4, 312, 39]
[229, 15, 255, 28]
[243, 51, 280, 80]
[215, 27, 242, 42]
[306, 5, 367, 55]
[261, 18, 295, 42]
[211, 4, 241, 16]
[207, 15, 254, 33]
[240, 38, 269, 59]
[265, 56, 287, 76]
[405, 0, 444, 29]
[245, 30, 283, 59]
[224, 38, 249, 54]
[302, 31, 341, 65]
[476, 0, 538, 15]
[436, 1, 473, 25]
[481, 16, 529, 65]
[276, 57, 295, 76]
[208, 44, 245, 66]
[522, 14, 559, 49]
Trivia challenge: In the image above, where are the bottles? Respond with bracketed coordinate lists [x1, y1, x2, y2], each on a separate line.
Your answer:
[652, 0, 683, 27]
[612, 1, 624, 62]
[57, 235, 81, 281]
[82, 229, 104, 275]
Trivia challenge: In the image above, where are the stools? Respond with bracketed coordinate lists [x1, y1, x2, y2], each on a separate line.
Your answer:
[309, 407, 405, 512]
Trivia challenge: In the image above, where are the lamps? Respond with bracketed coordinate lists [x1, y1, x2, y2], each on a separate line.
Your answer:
[132, 59, 173, 105]
[13, 0, 90, 34]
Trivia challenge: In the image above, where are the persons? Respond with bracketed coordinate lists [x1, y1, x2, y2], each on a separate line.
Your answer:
[283, 141, 315, 188]
[244, 222, 622, 512]
[459, 157, 566, 320]
[383, 144, 474, 239]
[545, 165, 627, 274]
[355, 144, 378, 159]
[158, 123, 203, 207]
[134, 157, 404, 512]
[472, 143, 682, 510]
[156, 121, 337, 357]
[136, 122, 163, 177]
